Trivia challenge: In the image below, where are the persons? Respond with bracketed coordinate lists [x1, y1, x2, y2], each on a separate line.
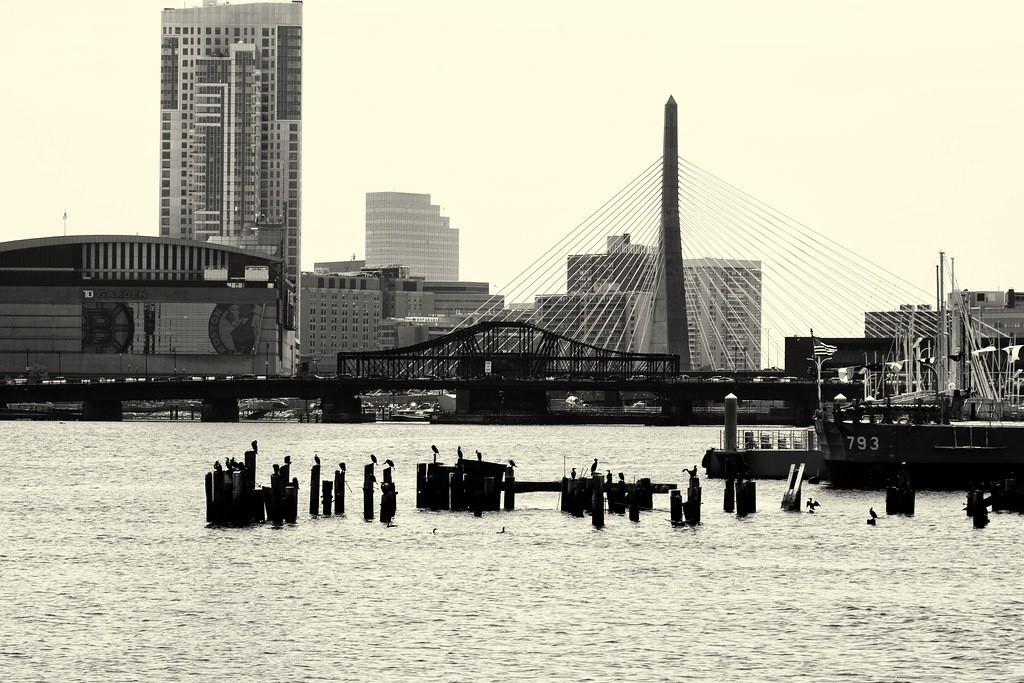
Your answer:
[850, 398, 860, 409]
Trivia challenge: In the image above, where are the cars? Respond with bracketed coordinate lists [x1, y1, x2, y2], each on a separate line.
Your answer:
[706, 375, 734, 382]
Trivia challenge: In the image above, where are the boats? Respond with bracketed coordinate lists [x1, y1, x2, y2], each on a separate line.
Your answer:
[391, 409, 432, 421]
[814, 251, 1024, 484]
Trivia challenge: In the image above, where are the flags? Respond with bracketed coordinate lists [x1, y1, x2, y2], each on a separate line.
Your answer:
[813, 338, 837, 355]
[885, 361, 905, 374]
[839, 366, 855, 381]
[946, 351, 963, 362]
[917, 357, 935, 365]
[864, 362, 880, 370]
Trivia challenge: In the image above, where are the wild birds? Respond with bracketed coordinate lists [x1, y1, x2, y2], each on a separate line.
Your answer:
[571, 468, 577, 479]
[869, 507, 879, 518]
[458, 446, 463, 459]
[273, 456, 292, 473]
[292, 477, 299, 489]
[508, 459, 517, 468]
[475, 450, 481, 460]
[371, 454, 378, 465]
[806, 498, 820, 509]
[339, 462, 346, 471]
[368, 474, 398, 495]
[619, 473, 624, 481]
[682, 465, 697, 479]
[591, 458, 598, 475]
[606, 470, 612, 483]
[213, 439, 259, 472]
[314, 454, 320, 465]
[431, 444, 439, 454]
[382, 459, 395, 470]
